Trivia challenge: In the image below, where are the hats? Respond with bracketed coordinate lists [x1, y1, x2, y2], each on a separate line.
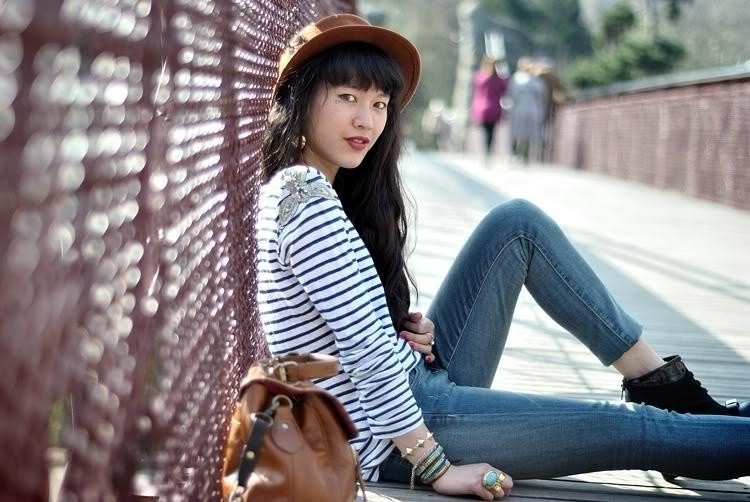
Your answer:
[271, 12, 423, 115]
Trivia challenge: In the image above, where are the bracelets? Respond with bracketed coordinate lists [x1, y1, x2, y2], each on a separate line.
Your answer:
[401, 431, 434, 460]
[410, 442, 454, 492]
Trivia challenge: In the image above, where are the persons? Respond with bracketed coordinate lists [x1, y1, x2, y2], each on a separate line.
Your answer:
[521, 61, 567, 168]
[433, 111, 454, 153]
[468, 52, 510, 158]
[253, 12, 750, 502]
[503, 56, 550, 168]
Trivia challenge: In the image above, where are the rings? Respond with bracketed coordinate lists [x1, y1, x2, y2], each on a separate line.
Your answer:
[490, 483, 502, 494]
[497, 471, 506, 482]
[482, 471, 498, 491]
[427, 334, 435, 346]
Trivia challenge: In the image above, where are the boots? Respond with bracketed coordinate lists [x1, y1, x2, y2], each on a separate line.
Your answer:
[623, 352, 750, 481]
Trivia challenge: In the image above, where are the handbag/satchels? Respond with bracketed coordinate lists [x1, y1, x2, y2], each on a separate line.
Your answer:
[221, 347, 365, 497]
